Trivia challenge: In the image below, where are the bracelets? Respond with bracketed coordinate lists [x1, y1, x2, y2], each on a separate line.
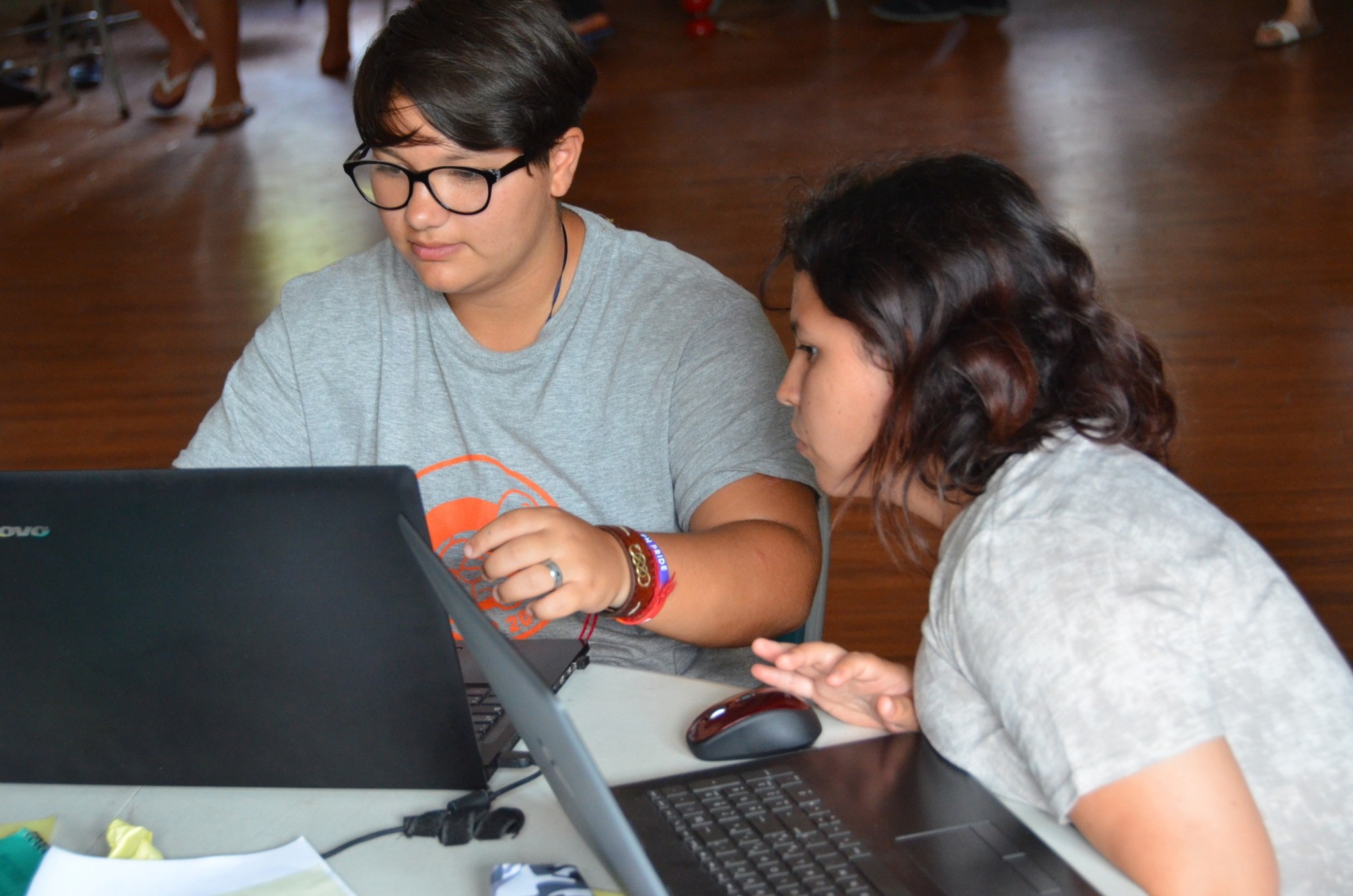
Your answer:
[595, 523, 676, 626]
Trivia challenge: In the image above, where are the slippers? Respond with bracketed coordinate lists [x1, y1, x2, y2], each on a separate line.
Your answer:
[197, 101, 254, 132]
[149, 60, 194, 109]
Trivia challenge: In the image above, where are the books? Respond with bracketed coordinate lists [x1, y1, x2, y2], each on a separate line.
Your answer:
[0, 827, 355, 896]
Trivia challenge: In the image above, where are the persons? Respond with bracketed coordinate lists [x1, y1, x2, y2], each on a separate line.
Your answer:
[173, 0, 830, 693]
[318, 0, 353, 81]
[132, 0, 253, 136]
[751, 151, 1353, 896]
[1251, 0, 1324, 49]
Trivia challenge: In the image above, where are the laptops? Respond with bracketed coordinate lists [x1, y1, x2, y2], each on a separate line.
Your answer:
[1, 466, 589, 794]
[394, 511, 1152, 896]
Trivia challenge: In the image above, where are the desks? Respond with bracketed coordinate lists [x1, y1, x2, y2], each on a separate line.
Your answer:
[1, 664, 1149, 896]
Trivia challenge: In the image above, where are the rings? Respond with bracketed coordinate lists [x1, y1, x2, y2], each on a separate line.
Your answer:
[544, 559, 563, 592]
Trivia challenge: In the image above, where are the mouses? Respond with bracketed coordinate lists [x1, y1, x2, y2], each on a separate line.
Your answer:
[685, 685, 822, 761]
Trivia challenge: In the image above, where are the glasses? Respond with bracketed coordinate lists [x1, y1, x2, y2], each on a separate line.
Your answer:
[343, 139, 556, 216]
